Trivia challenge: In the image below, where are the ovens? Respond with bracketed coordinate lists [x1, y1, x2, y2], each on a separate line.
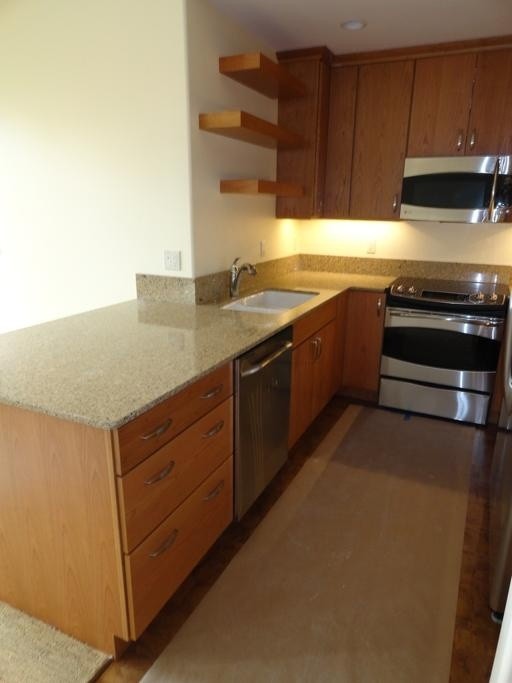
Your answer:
[377, 306, 505, 428]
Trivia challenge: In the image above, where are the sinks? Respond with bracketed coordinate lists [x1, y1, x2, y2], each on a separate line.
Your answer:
[221, 286, 318, 314]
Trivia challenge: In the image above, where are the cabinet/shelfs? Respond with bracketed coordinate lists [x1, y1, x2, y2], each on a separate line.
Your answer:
[1, 361, 234, 661]
[342, 294, 386, 409]
[275, 58, 331, 220]
[233, 320, 291, 523]
[323, 48, 415, 220]
[199, 52, 314, 196]
[293, 291, 353, 455]
[406, 34, 511, 157]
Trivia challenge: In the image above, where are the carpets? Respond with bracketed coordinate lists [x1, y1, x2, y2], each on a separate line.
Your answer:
[0, 600, 111, 683]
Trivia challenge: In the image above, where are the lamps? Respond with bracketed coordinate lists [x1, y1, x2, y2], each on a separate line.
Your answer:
[341, 19, 367, 31]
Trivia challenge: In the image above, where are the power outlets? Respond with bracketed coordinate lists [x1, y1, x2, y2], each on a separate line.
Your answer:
[164, 248, 181, 271]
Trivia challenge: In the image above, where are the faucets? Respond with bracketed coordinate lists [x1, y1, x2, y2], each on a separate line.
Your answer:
[229, 255, 257, 300]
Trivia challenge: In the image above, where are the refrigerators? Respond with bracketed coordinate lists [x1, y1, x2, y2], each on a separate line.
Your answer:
[490, 301, 511, 620]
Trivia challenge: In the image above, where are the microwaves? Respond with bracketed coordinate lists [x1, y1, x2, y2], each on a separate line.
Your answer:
[399, 154, 512, 222]
